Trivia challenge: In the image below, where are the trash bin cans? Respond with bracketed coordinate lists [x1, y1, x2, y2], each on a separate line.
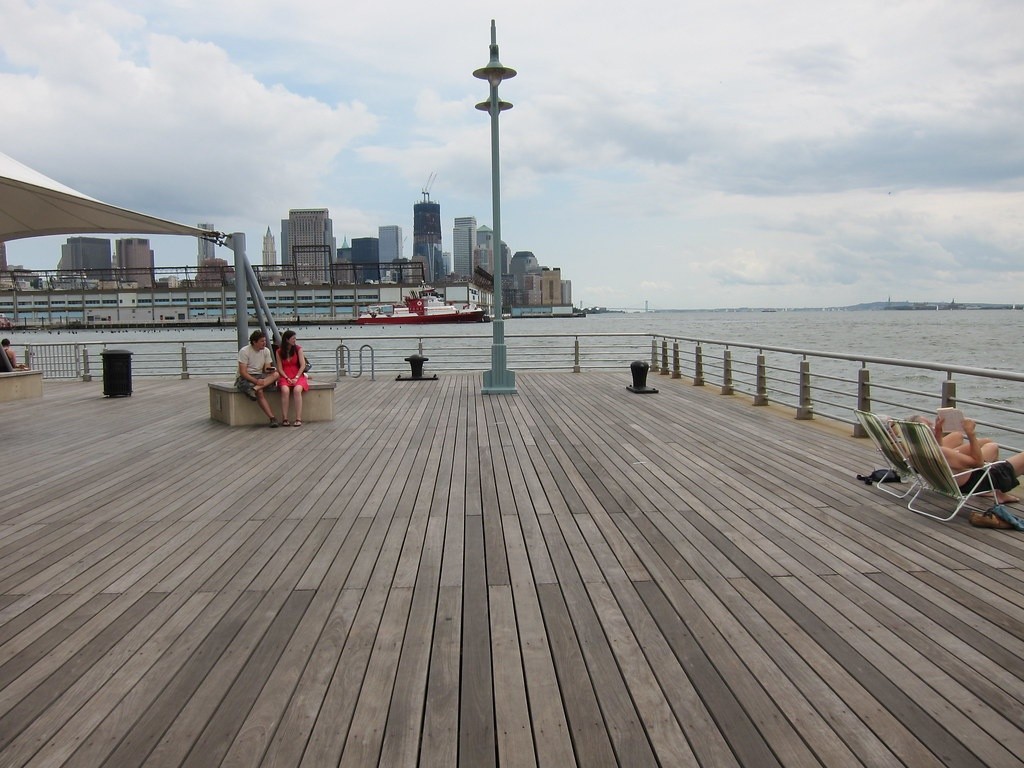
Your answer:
[100, 348, 134, 399]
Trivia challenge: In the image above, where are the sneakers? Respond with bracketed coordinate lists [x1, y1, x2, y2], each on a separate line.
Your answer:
[270, 419, 279, 426]
[245, 387, 257, 401]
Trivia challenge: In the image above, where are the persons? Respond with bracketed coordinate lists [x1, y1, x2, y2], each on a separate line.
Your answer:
[0, 338, 17, 370]
[233, 329, 280, 428]
[6, 318, 10, 325]
[269, 332, 288, 374]
[902, 409, 1023, 511]
[274, 330, 308, 427]
[876, 410, 993, 478]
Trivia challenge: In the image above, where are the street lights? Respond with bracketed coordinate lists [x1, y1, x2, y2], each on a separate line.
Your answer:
[473, 19, 518, 394]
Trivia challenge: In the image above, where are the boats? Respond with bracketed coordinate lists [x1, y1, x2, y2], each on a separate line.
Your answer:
[357, 279, 487, 323]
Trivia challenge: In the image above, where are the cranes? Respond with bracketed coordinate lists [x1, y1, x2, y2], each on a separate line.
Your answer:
[422, 170, 437, 206]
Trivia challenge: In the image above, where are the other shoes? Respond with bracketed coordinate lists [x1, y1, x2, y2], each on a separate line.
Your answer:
[283, 419, 290, 425]
[969, 511, 1013, 529]
[294, 420, 302, 427]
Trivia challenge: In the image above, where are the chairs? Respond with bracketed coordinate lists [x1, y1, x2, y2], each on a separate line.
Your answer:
[854, 407, 1007, 523]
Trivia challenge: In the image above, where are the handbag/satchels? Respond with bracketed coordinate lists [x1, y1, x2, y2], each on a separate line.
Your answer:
[872, 469, 900, 482]
[295, 344, 312, 373]
